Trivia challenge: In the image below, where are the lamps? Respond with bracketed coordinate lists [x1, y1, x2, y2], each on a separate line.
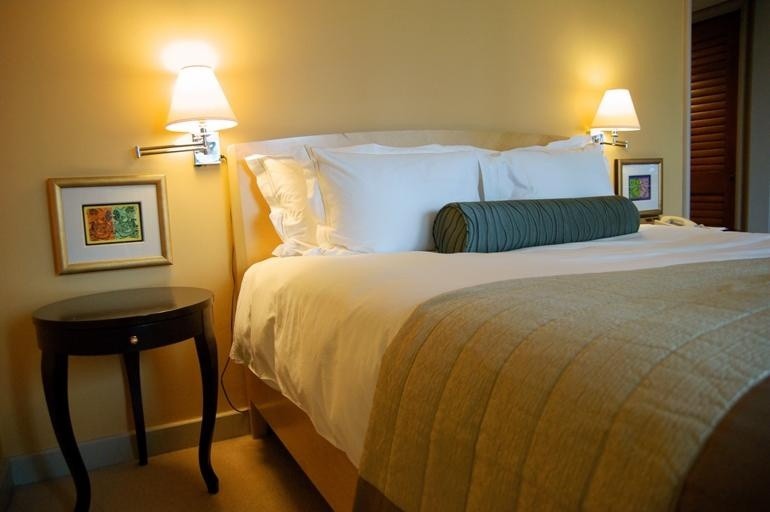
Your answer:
[591, 88, 641, 148]
[135, 64, 239, 167]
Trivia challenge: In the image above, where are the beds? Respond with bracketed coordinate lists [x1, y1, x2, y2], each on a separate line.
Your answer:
[226, 130, 769, 510]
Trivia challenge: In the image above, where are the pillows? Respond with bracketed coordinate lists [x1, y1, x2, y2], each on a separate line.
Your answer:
[301, 143, 481, 256]
[476, 135, 615, 202]
[244, 151, 326, 258]
[431, 195, 640, 254]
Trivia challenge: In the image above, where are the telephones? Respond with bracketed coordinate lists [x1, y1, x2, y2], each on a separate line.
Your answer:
[654, 216, 698, 228]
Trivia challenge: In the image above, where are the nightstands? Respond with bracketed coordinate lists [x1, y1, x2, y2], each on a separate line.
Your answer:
[32, 286, 219, 511]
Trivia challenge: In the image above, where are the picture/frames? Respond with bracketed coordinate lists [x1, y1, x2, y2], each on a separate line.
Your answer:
[616, 158, 663, 217]
[47, 174, 173, 275]
